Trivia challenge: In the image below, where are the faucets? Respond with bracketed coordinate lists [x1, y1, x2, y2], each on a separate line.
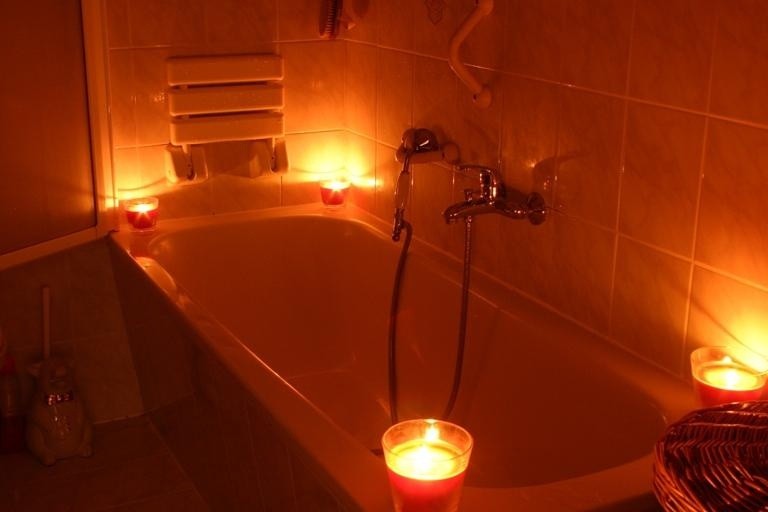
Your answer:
[444, 163, 545, 226]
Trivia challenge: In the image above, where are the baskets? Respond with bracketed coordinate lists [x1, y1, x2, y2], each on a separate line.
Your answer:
[651, 401, 767, 511]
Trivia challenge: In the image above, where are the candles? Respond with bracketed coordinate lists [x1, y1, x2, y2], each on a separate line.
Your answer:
[320, 177, 350, 209]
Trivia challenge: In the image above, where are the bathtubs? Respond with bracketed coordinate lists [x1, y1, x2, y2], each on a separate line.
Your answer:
[110, 201, 702, 512]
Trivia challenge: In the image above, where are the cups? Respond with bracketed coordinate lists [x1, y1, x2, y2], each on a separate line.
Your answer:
[319, 176, 351, 208]
